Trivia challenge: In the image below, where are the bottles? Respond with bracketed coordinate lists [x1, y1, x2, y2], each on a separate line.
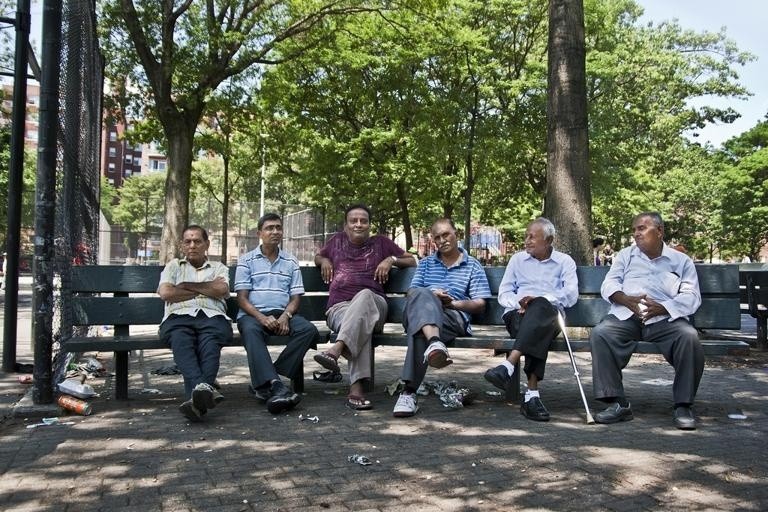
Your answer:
[57, 394, 93, 417]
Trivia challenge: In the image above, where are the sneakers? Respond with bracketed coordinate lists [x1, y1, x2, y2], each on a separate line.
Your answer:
[192, 383, 214, 415]
[393, 391, 419, 416]
[267, 392, 301, 414]
[181, 399, 198, 419]
[423, 341, 453, 369]
[249, 384, 265, 402]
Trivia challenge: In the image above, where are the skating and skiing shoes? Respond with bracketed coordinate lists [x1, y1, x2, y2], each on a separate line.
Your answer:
[673, 407, 696, 429]
[594, 401, 633, 423]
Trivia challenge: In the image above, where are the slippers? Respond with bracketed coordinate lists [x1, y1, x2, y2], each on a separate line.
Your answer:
[314, 351, 340, 371]
[346, 395, 373, 409]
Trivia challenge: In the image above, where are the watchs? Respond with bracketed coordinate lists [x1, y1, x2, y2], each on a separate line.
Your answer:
[391, 256, 398, 263]
[284, 310, 292, 319]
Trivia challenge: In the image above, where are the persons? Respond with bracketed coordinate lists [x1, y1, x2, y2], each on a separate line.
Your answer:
[603, 244, 613, 266]
[157, 225, 234, 422]
[741, 252, 751, 264]
[485, 218, 578, 421]
[590, 213, 705, 429]
[314, 203, 417, 410]
[236, 213, 319, 413]
[592, 238, 604, 265]
[393, 218, 491, 417]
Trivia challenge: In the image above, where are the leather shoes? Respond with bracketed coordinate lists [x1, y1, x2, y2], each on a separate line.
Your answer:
[484, 365, 512, 390]
[520, 397, 550, 420]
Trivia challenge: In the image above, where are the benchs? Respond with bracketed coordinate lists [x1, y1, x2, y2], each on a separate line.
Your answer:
[61, 260, 744, 402]
[739, 264, 768, 347]
